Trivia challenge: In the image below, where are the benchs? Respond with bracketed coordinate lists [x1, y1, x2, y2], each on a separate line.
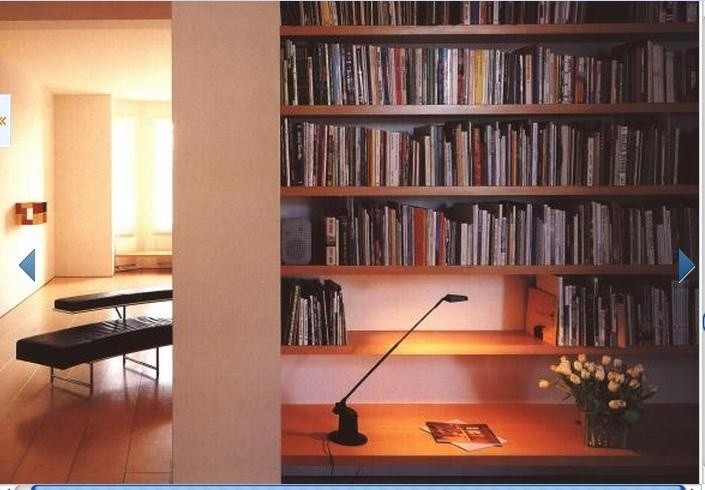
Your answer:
[17, 313, 173, 395]
[54, 284, 174, 319]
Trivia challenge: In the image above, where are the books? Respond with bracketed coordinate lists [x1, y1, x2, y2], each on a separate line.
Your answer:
[536, 120, 699, 187]
[538, 201, 700, 269]
[419, 416, 506, 451]
[493, 1, 698, 26]
[280, 117, 539, 189]
[515, 42, 698, 106]
[280, 41, 533, 105]
[310, 199, 535, 265]
[282, 279, 348, 347]
[282, 2, 489, 26]
[426, 419, 503, 446]
[525, 277, 700, 351]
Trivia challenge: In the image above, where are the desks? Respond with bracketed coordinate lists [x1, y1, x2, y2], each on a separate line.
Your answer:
[281, 400, 699, 482]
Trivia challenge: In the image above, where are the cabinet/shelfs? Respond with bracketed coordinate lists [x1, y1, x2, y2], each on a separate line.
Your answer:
[278, 2, 698, 399]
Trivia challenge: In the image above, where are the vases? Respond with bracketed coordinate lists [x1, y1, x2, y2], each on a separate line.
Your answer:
[582, 409, 632, 446]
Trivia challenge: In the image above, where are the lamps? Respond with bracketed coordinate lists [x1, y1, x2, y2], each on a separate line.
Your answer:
[328, 293, 469, 445]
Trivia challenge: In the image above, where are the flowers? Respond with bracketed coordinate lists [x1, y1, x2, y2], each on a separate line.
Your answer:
[534, 354, 662, 445]
